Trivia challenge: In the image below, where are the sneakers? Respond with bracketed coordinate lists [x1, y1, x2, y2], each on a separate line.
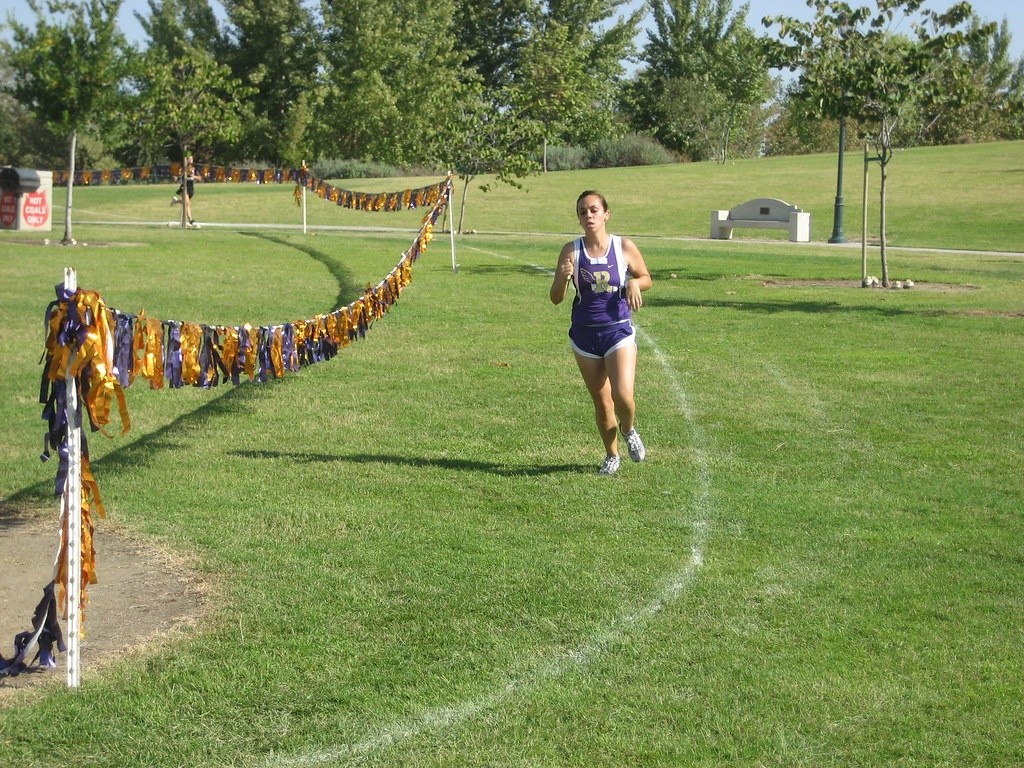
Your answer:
[599, 455, 621, 475]
[619, 423, 645, 463]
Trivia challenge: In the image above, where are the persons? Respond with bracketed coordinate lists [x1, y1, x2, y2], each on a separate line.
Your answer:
[549, 190, 653, 476]
[171, 155, 202, 227]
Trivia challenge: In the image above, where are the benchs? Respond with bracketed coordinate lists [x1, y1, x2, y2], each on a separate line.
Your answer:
[710, 197, 810, 242]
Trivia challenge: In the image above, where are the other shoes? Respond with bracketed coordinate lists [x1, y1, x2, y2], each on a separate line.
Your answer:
[169, 197, 178, 207]
[191, 222, 201, 229]
